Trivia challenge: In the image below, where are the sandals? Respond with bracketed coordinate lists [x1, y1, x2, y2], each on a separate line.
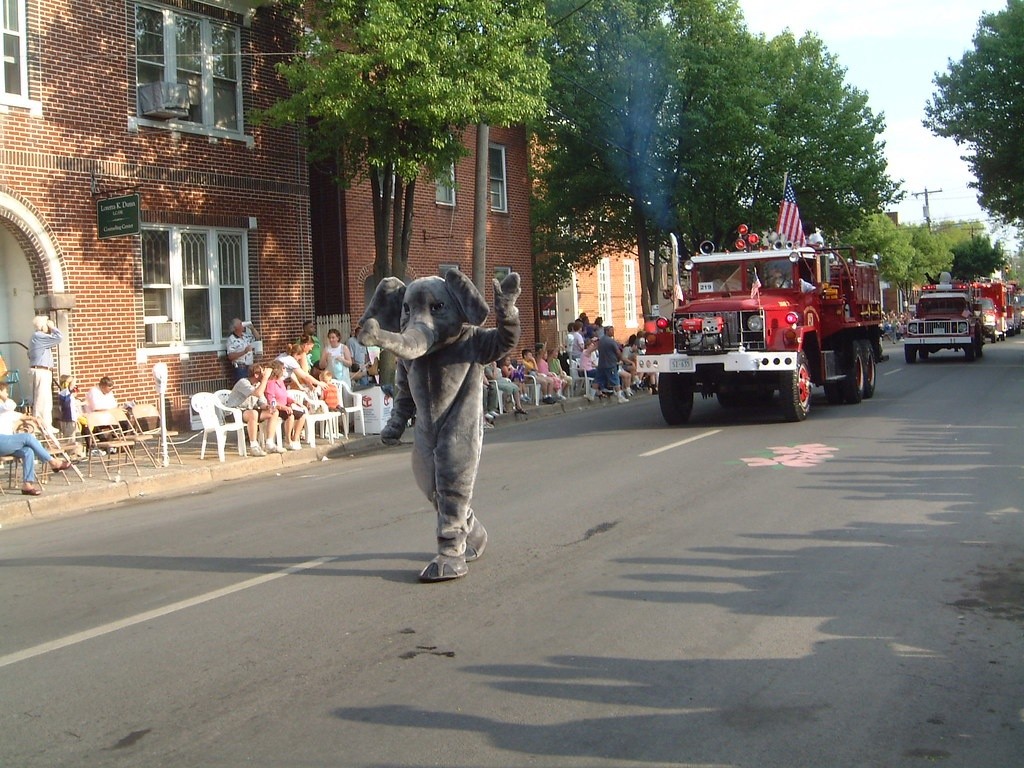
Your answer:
[515, 407, 528, 414]
[595, 393, 608, 398]
[497, 405, 509, 413]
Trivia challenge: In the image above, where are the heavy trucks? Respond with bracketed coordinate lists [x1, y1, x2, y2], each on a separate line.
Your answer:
[904, 277, 1024, 364]
[635, 224, 889, 426]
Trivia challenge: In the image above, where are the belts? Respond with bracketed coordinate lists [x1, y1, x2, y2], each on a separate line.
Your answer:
[31, 366, 53, 370]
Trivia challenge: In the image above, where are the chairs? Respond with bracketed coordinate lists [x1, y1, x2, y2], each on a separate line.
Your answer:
[0, 350, 646, 493]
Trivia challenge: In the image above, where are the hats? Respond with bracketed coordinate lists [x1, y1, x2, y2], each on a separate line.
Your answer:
[32, 315, 49, 331]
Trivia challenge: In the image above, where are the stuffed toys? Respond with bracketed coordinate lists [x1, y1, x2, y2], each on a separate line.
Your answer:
[354, 269, 523, 581]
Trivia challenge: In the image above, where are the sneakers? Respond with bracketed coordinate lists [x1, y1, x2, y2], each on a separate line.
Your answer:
[110, 448, 117, 453]
[623, 387, 634, 396]
[266, 444, 287, 452]
[617, 396, 629, 403]
[285, 441, 303, 450]
[490, 411, 500, 416]
[584, 394, 594, 404]
[48, 427, 59, 433]
[248, 445, 266, 457]
[484, 413, 493, 419]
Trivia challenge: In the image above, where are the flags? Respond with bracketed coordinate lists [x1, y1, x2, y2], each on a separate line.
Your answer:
[776, 175, 807, 248]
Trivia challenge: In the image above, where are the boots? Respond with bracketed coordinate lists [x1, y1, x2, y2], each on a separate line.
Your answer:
[651, 385, 658, 394]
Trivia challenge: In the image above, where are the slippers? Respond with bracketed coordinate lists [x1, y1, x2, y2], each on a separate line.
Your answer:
[52, 461, 71, 472]
[22, 488, 41, 495]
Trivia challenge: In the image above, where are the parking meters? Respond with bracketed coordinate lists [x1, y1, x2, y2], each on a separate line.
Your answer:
[152, 362, 168, 467]
[566, 334, 574, 398]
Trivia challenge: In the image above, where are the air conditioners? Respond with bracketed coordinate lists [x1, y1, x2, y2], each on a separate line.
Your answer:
[144, 81, 188, 119]
[144, 323, 183, 347]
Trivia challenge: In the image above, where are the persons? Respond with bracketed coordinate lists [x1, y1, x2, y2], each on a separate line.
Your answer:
[28, 314, 63, 435]
[59, 373, 106, 458]
[87, 376, 130, 455]
[776, 265, 815, 293]
[222, 314, 381, 456]
[0, 380, 71, 497]
[479, 312, 661, 428]
[877, 307, 919, 345]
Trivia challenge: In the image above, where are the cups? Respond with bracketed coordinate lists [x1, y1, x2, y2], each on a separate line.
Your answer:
[242, 321, 252, 327]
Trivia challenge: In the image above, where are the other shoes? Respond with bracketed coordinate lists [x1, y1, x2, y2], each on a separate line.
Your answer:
[86, 448, 107, 458]
[543, 396, 557, 404]
[557, 394, 567, 400]
[520, 394, 531, 402]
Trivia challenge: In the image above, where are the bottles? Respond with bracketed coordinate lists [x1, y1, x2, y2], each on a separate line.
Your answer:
[269, 395, 277, 414]
